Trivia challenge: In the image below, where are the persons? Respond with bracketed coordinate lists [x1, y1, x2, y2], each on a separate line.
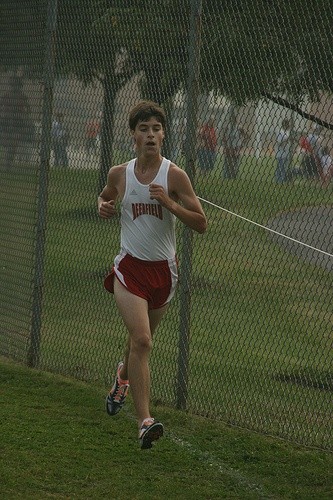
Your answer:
[124, 105, 250, 180]
[0, 77, 99, 167]
[274, 119, 333, 184]
[98, 100, 207, 449]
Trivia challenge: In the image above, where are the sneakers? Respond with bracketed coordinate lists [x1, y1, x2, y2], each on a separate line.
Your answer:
[105, 361, 131, 416]
[137, 417, 164, 450]
